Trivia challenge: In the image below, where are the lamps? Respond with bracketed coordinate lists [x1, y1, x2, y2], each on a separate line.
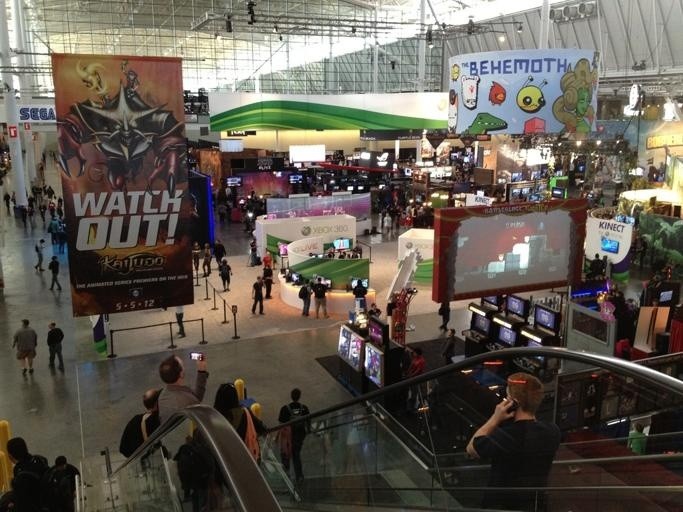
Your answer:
[497, 33, 505, 43]
[245, 0, 257, 25]
[468, 19, 474, 35]
[391, 61, 395, 69]
[663, 97, 682, 122]
[517, 23, 523, 33]
[273, 17, 282, 41]
[352, 26, 356, 34]
[426, 26, 434, 48]
[223, 9, 233, 32]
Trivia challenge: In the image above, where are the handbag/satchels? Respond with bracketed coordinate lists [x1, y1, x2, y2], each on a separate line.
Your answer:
[35, 244, 40, 253]
[243, 406, 261, 468]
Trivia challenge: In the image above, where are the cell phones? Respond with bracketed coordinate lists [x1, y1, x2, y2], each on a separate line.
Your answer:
[506, 400, 519, 411]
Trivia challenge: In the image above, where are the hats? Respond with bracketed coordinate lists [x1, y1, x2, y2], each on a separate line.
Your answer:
[21, 319, 29, 324]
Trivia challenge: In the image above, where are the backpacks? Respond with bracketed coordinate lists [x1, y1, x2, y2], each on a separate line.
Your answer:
[222, 266, 229, 275]
[299, 287, 308, 299]
[289, 414, 307, 441]
[314, 284, 326, 299]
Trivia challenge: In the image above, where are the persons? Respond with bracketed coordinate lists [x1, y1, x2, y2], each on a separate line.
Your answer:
[427, 375, 451, 433]
[438, 289, 456, 364]
[403, 350, 425, 404]
[118, 355, 314, 512]
[1, 180, 438, 376]
[586, 253, 605, 278]
[466, 373, 562, 511]
[1, 437, 81, 512]
[627, 424, 648, 455]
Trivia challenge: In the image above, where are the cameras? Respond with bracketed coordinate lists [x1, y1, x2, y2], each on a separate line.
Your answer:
[190, 352, 203, 360]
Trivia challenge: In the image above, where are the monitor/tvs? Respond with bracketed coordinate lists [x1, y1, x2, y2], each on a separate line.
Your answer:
[511, 164, 585, 203]
[659, 290, 672, 303]
[315, 278, 332, 289]
[528, 340, 546, 362]
[291, 273, 301, 282]
[498, 327, 518, 347]
[288, 174, 304, 184]
[351, 277, 369, 290]
[505, 295, 531, 319]
[535, 304, 562, 333]
[600, 238, 619, 253]
[471, 314, 491, 335]
[337, 326, 351, 363]
[348, 333, 362, 372]
[365, 343, 385, 389]
[225, 177, 243, 187]
[334, 239, 350, 250]
[482, 295, 504, 306]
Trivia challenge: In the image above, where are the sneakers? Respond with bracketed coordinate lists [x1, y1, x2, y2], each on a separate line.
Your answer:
[21, 368, 28, 374]
[29, 368, 34, 373]
